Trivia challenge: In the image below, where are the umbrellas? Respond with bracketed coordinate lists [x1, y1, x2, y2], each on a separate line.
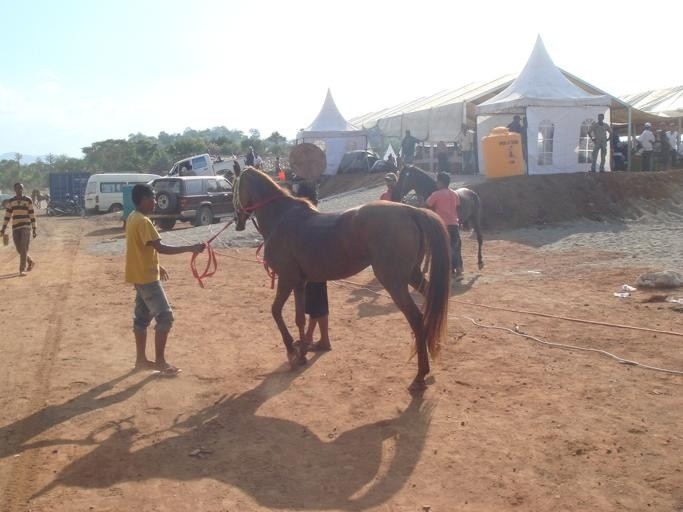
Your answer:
[289, 142, 326, 180]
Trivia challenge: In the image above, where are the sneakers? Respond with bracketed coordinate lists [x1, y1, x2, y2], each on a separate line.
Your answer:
[135, 355, 183, 378]
[19, 258, 36, 276]
[452, 270, 465, 283]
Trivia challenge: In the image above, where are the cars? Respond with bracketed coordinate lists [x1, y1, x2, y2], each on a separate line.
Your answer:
[0, 193, 12, 210]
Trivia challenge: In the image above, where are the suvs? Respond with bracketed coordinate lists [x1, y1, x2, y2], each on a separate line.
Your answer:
[147, 175, 232, 232]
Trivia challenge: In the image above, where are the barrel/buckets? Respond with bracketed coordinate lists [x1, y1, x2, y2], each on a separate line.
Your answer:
[480, 125, 525, 177]
[279, 171, 286, 181]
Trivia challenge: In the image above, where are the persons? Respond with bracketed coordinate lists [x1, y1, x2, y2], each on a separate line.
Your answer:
[0, 184, 38, 276]
[215, 146, 280, 179]
[587, 112, 612, 173]
[638, 122, 655, 171]
[460, 122, 474, 173]
[666, 123, 680, 169]
[294, 175, 318, 207]
[31, 192, 37, 207]
[438, 141, 449, 173]
[401, 130, 429, 164]
[379, 172, 397, 201]
[124, 183, 205, 375]
[418, 172, 465, 282]
[506, 115, 522, 134]
[657, 122, 670, 170]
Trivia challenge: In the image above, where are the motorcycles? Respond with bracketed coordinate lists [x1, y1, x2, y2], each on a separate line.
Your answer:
[43, 191, 85, 218]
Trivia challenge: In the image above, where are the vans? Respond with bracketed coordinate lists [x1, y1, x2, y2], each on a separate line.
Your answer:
[82, 172, 160, 214]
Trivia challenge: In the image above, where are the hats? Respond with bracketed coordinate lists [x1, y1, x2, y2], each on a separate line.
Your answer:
[643, 122, 653, 128]
[384, 172, 397, 183]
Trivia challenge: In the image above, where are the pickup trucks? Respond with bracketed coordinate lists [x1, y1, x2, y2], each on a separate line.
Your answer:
[162, 152, 248, 176]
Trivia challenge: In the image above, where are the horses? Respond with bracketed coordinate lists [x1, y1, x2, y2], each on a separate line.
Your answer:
[389, 158, 485, 272]
[34, 189, 50, 210]
[231, 158, 452, 392]
